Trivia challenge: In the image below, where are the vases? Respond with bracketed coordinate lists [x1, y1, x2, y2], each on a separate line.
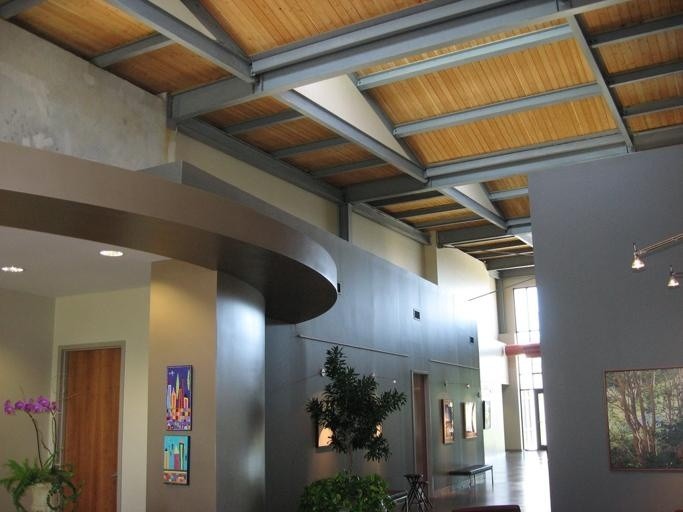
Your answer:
[25, 483, 60, 512]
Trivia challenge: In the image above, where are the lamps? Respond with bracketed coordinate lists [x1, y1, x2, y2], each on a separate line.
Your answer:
[666, 264, 683, 287]
[631, 234, 682, 270]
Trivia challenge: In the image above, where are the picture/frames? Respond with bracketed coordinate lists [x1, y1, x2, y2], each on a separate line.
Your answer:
[441, 398, 491, 445]
[604, 364, 682, 472]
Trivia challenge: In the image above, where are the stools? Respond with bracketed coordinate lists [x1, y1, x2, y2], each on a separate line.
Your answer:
[402, 474, 433, 512]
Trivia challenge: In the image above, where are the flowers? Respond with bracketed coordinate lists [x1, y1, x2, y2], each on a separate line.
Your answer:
[0, 394, 86, 512]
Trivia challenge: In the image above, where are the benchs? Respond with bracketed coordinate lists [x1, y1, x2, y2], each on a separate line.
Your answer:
[448, 465, 493, 487]
[382, 488, 408, 512]
